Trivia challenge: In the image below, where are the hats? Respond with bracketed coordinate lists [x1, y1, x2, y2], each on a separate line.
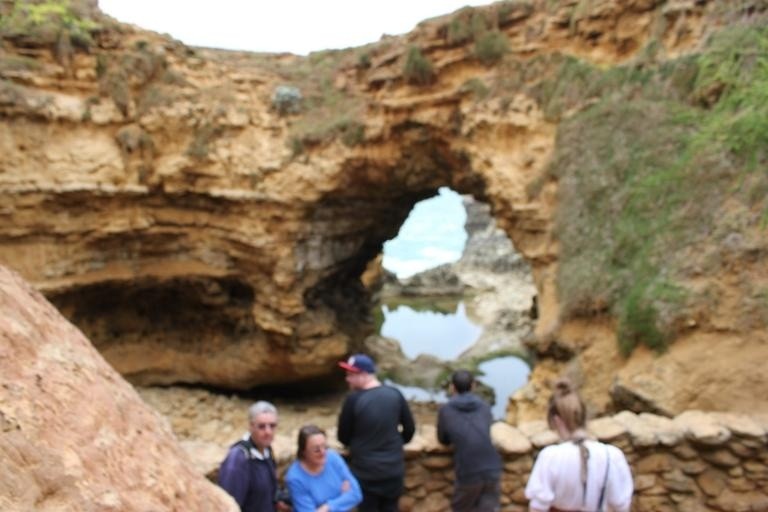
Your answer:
[338, 354, 375, 374]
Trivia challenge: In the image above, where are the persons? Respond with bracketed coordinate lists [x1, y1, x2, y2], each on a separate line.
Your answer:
[436, 370, 505, 512]
[337, 354, 417, 512]
[217, 397, 293, 511]
[524, 379, 636, 512]
[285, 423, 366, 512]
